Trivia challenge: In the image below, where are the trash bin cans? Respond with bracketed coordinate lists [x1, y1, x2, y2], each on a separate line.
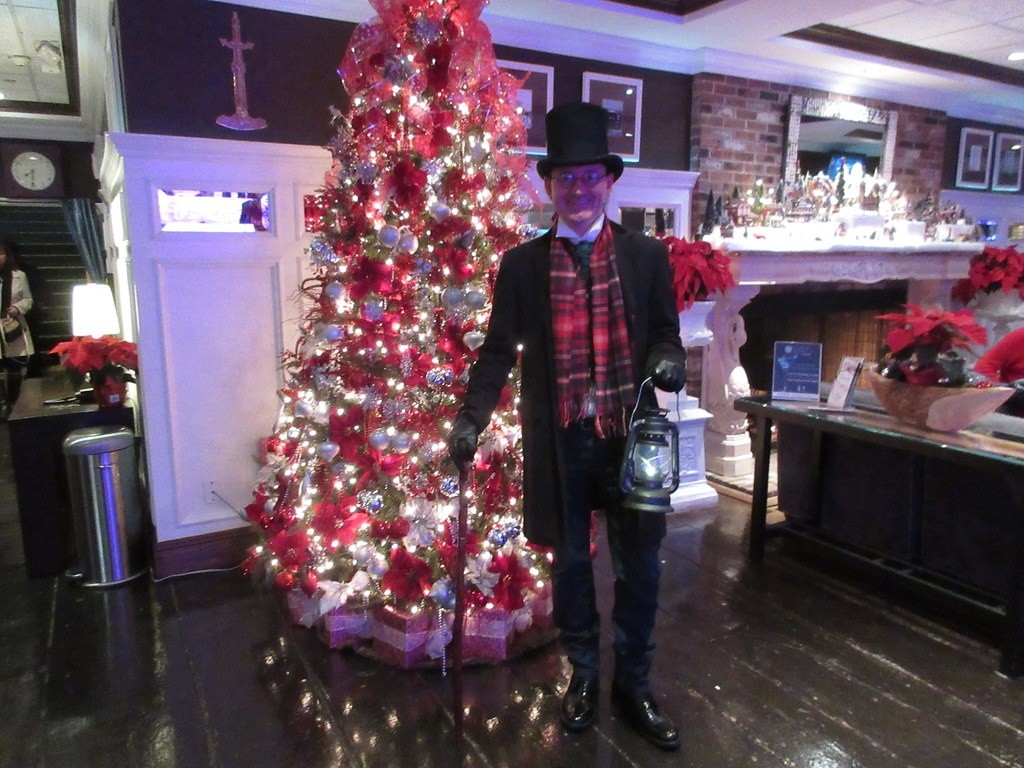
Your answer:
[62, 425, 147, 589]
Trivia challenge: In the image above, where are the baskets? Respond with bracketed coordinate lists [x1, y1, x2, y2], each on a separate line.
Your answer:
[867, 357, 1017, 431]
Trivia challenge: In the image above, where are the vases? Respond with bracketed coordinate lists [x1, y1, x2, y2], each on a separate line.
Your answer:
[974, 289, 1024, 343]
[681, 303, 716, 349]
[863, 366, 1016, 432]
[93, 376, 128, 406]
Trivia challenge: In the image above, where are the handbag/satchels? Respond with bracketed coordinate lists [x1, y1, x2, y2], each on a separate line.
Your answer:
[0, 314, 31, 365]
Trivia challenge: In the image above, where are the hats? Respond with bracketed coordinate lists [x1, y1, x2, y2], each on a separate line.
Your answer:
[536, 101, 625, 185]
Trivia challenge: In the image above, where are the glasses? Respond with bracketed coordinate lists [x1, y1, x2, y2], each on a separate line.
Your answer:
[547, 171, 612, 187]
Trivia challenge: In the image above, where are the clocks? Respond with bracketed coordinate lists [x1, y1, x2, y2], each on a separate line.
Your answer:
[11, 152, 56, 191]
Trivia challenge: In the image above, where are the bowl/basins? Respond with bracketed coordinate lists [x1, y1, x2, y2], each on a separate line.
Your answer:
[868, 364, 1016, 431]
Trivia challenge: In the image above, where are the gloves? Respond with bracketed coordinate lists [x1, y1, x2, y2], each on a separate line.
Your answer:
[648, 346, 687, 394]
[444, 416, 479, 464]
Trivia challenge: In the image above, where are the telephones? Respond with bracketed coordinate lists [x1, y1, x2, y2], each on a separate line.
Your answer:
[74, 388, 96, 404]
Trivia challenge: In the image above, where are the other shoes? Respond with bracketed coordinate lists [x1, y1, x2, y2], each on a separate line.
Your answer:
[2, 402, 15, 418]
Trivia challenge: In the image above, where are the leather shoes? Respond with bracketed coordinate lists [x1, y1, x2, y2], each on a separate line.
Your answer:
[614, 696, 683, 749]
[560, 671, 600, 728]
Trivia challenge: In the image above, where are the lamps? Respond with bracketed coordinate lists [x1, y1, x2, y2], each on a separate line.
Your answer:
[71, 283, 121, 404]
[618, 377, 681, 514]
[35, 39, 62, 74]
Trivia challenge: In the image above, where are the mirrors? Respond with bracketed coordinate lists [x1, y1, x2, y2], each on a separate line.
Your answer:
[518, 203, 556, 229]
[156, 187, 270, 234]
[781, 95, 900, 216]
[618, 202, 681, 240]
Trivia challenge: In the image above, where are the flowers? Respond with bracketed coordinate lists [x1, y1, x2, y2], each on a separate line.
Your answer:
[874, 305, 990, 389]
[654, 229, 740, 313]
[950, 243, 1024, 308]
[49, 334, 141, 384]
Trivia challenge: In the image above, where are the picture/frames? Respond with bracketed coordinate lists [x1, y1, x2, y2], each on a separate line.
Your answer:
[955, 126, 995, 189]
[582, 72, 643, 162]
[495, 60, 555, 155]
[992, 134, 1024, 192]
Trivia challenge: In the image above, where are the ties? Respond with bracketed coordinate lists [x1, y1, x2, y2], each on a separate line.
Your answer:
[560, 235, 597, 291]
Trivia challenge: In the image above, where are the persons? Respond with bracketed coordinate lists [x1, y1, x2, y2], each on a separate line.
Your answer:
[0, 244, 34, 406]
[974, 325, 1024, 386]
[445, 100, 690, 748]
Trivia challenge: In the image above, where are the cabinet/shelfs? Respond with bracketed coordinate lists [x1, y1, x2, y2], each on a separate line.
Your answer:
[7, 378, 150, 576]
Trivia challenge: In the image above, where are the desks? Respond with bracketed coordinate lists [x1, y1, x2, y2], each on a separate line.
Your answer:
[734, 397, 1023, 678]
